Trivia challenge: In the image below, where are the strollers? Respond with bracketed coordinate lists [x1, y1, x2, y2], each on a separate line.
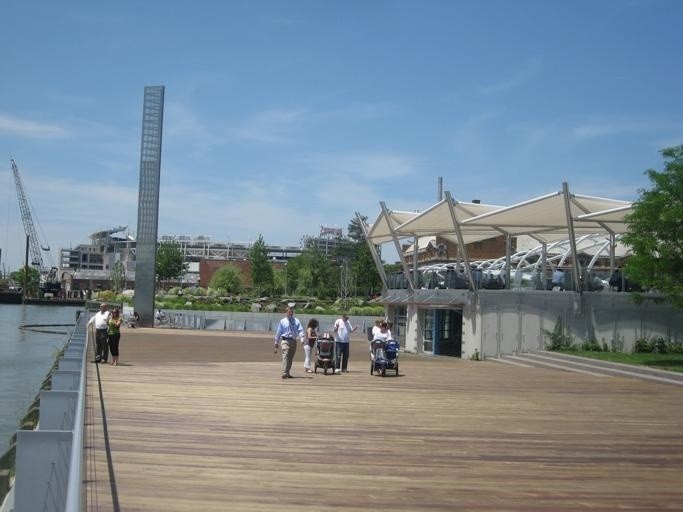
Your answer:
[314, 331, 336, 377]
[366, 340, 400, 378]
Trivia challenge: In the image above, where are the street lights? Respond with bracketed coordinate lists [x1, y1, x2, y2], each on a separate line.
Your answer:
[178, 261, 189, 292]
[338, 264, 343, 297]
[283, 262, 288, 298]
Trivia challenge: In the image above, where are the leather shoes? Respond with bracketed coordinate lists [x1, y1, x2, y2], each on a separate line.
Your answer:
[92, 360, 118, 365]
[305, 369, 313, 373]
[283, 374, 292, 378]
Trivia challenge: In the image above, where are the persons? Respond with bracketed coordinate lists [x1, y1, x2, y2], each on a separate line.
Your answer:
[272, 306, 305, 378]
[372, 321, 390, 376]
[156, 310, 162, 321]
[176, 287, 183, 296]
[86, 303, 109, 364]
[374, 320, 392, 339]
[590, 273, 603, 290]
[304, 317, 319, 373]
[333, 313, 358, 373]
[131, 311, 140, 327]
[551, 267, 564, 291]
[371, 319, 381, 337]
[107, 308, 123, 366]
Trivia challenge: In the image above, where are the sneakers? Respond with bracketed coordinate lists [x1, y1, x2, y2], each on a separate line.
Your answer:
[337, 368, 348, 373]
